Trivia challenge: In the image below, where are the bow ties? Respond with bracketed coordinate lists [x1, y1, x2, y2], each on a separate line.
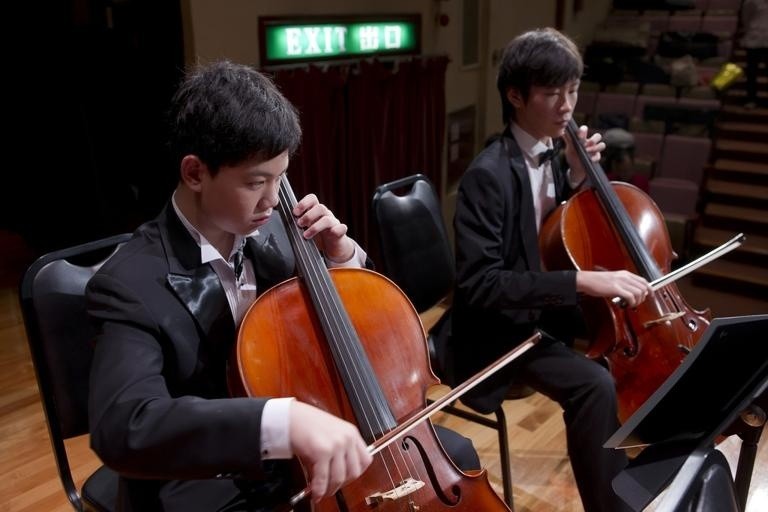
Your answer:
[539, 141, 564, 167]
[233, 246, 246, 285]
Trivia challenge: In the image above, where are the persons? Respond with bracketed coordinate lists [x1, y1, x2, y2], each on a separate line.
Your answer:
[85, 61, 481, 512]
[451, 26, 656, 512]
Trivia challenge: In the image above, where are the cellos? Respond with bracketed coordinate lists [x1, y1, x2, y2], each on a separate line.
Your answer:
[538, 117, 728, 443]
[226, 172, 511, 512]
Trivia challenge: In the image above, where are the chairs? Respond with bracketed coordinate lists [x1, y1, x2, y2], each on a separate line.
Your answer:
[370, 175, 533, 511]
[18, 233, 134, 512]
[634, 448, 742, 511]
[573, 0, 743, 266]
